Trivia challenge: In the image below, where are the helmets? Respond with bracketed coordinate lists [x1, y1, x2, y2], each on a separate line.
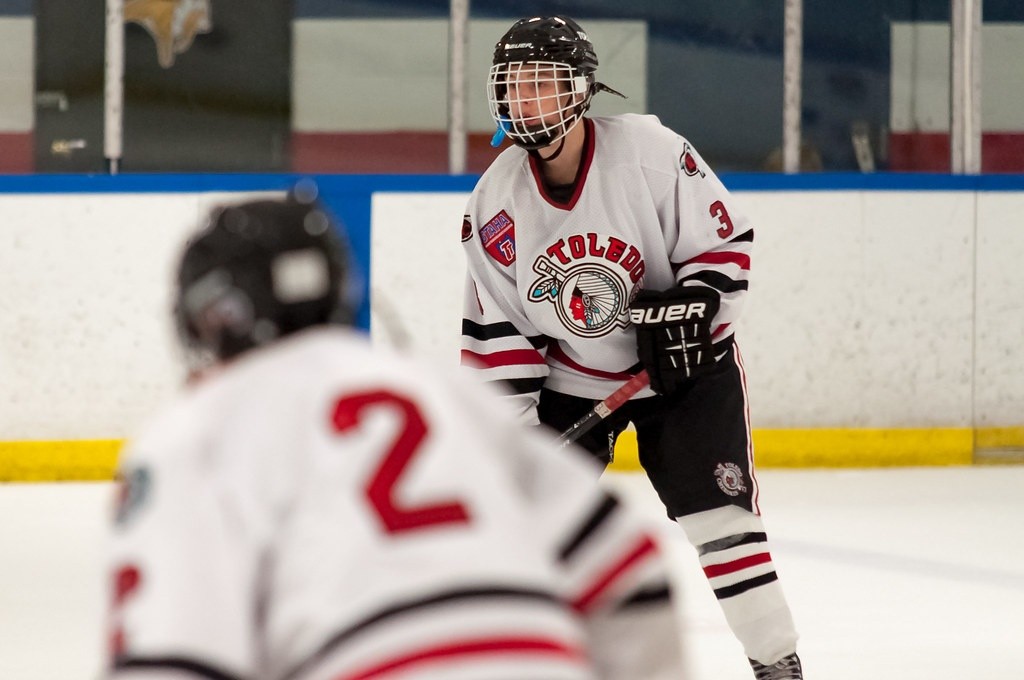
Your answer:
[172, 199, 345, 351]
[487, 14, 629, 150]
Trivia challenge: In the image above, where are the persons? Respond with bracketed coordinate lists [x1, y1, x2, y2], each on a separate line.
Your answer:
[460, 16, 803, 680]
[105, 199, 758, 680]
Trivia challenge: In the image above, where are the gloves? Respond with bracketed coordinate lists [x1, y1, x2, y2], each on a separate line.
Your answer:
[629, 287, 728, 403]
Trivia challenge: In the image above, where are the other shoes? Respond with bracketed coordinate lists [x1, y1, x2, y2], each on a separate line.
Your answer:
[749, 654, 802, 680]
